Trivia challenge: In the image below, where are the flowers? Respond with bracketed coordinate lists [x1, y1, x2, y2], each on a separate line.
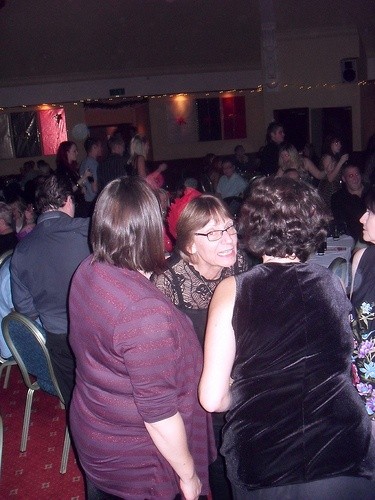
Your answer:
[347, 301, 375, 420]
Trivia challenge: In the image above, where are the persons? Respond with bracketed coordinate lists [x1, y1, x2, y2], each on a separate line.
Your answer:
[198, 173, 375, 500]
[151, 188, 260, 500]
[0, 125, 375, 250]
[67, 176, 218, 500]
[349, 189, 375, 440]
[0, 244, 47, 363]
[9, 174, 94, 407]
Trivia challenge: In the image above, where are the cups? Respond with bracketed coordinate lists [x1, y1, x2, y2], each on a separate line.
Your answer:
[317, 240, 324, 253]
[324, 240, 327, 250]
[332, 225, 339, 241]
[87, 168, 94, 184]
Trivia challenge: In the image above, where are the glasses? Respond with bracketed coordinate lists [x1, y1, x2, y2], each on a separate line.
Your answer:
[192, 224, 239, 242]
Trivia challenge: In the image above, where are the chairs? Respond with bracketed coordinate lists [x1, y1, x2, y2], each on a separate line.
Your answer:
[0, 249, 78, 475]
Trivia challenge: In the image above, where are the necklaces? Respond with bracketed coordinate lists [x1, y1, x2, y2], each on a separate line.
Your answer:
[198, 271, 215, 296]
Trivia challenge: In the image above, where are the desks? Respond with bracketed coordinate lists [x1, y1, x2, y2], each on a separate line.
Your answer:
[311, 235, 354, 296]
